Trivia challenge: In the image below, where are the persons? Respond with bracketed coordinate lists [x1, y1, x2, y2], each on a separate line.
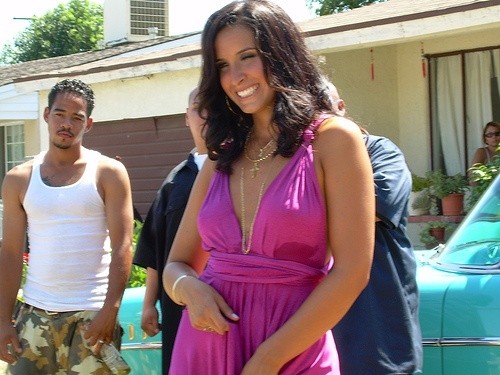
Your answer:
[323, 75, 424, 375]
[469, 121, 500, 186]
[131, 85, 207, 374]
[163, 1, 375, 375]
[0, 78, 134, 375]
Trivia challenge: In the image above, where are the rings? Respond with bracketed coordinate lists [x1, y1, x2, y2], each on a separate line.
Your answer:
[208, 326, 212, 329]
[98, 340, 104, 343]
[203, 327, 207, 330]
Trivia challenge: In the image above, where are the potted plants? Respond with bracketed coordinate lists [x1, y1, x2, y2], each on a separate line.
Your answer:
[407, 169, 465, 249]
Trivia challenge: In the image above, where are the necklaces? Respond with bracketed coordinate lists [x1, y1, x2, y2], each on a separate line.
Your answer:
[240, 130, 278, 255]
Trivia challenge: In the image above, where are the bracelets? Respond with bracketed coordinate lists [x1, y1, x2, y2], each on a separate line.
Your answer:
[172, 274, 198, 305]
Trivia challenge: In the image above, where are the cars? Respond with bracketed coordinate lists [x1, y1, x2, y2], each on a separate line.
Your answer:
[122, 173, 500, 375]
